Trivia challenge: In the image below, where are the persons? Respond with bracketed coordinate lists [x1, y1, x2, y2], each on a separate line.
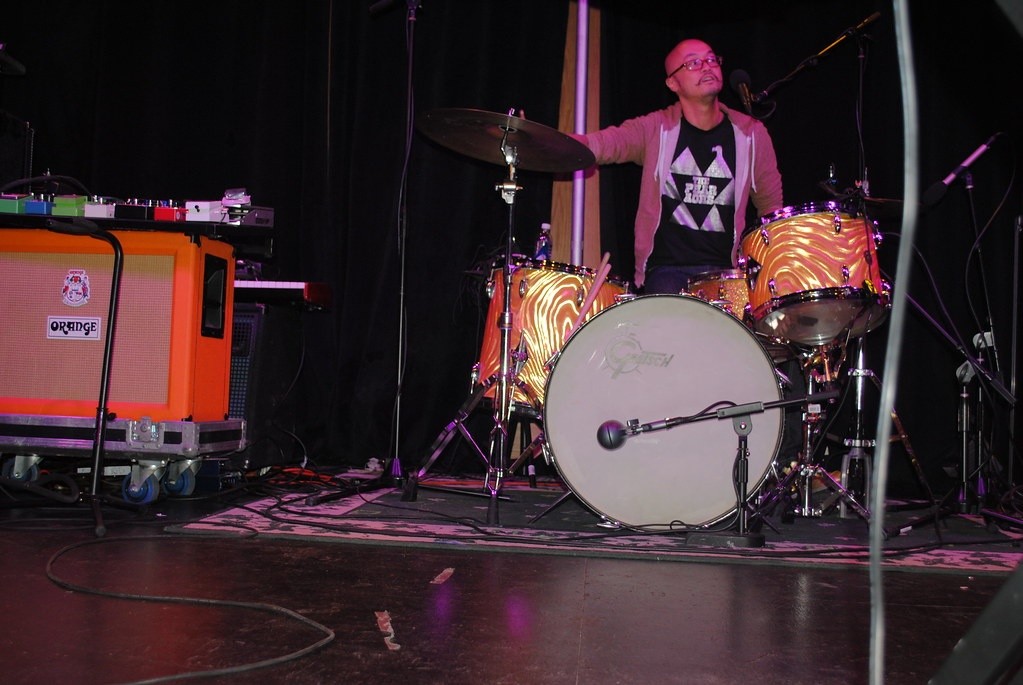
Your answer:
[518, 38, 843, 494]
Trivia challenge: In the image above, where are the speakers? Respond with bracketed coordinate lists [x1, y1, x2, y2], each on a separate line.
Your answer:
[0, 228, 234, 422]
[229, 302, 290, 449]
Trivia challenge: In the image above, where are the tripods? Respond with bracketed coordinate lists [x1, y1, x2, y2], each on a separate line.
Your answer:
[751, 13, 1022, 547]
[305, 1, 546, 526]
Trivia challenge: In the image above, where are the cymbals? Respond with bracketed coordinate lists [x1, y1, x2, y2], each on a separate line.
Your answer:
[419, 107, 597, 175]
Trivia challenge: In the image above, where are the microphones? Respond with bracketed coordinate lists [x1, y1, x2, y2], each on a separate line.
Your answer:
[597, 419, 666, 450]
[729, 70, 753, 115]
[941, 135, 996, 186]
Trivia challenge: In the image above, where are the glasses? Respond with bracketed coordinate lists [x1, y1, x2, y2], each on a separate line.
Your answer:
[669, 55, 724, 77]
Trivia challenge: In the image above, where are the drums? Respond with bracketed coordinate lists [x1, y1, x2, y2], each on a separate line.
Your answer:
[684, 266, 789, 366]
[540, 290, 812, 536]
[471, 255, 640, 423]
[736, 199, 891, 350]
